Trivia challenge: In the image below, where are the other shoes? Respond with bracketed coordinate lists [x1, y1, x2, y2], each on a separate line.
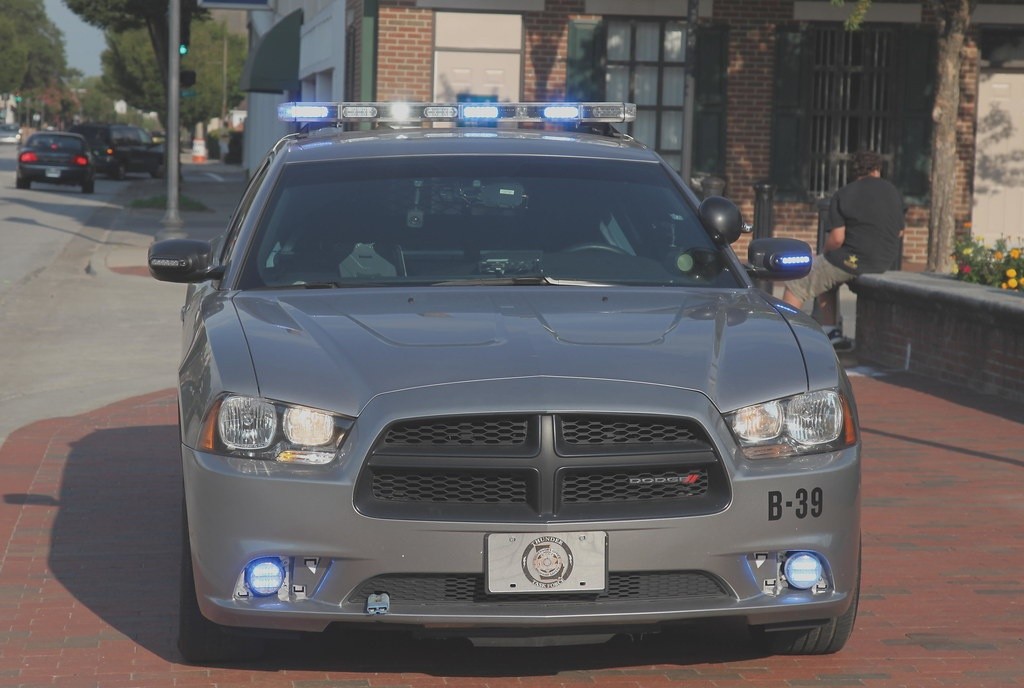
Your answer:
[825, 327, 846, 345]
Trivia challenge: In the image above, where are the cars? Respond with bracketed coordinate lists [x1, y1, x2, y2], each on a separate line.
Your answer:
[16, 131, 94, 194]
[146, 97, 865, 661]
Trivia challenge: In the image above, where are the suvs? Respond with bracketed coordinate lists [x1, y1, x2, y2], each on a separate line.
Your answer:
[68, 120, 165, 182]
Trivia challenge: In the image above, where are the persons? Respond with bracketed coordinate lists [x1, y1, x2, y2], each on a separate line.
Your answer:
[219, 122, 230, 164]
[64, 110, 73, 129]
[782, 150, 906, 344]
